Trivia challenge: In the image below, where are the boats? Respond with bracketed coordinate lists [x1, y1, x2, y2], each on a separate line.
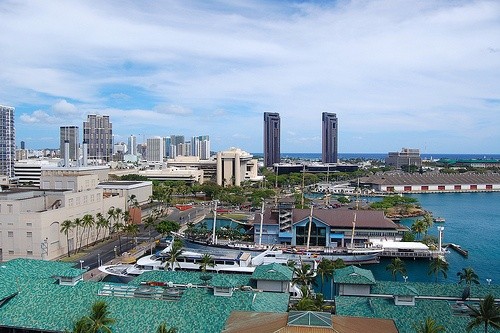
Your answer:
[77, 229, 449, 293]
